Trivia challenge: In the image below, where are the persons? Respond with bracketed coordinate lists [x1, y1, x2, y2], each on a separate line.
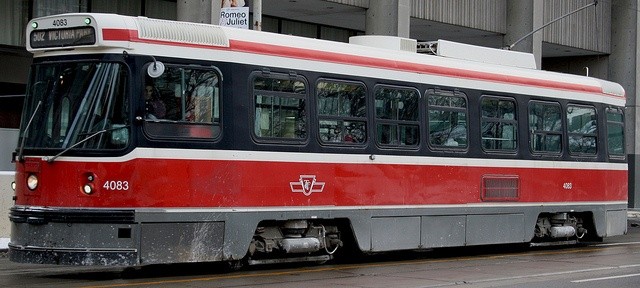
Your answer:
[145, 82, 166, 120]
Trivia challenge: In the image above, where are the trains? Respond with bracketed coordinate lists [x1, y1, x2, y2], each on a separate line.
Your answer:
[8, 13, 628, 269]
